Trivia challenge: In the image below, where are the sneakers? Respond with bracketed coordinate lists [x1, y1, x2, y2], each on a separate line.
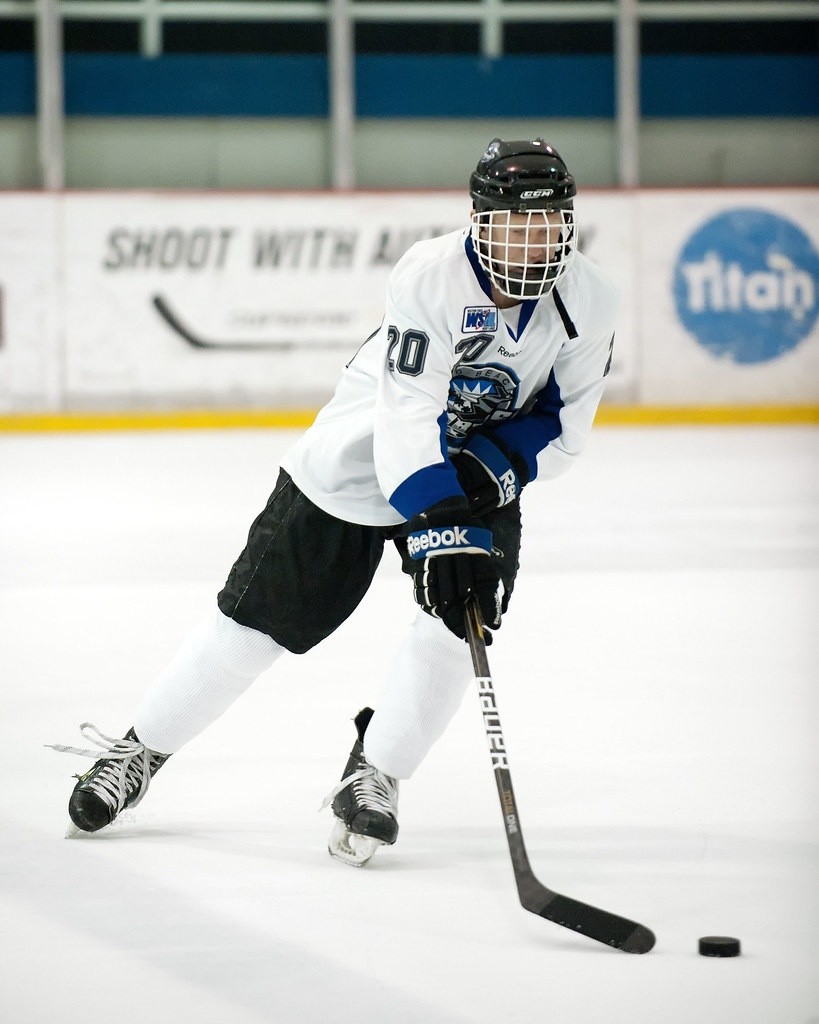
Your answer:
[45, 722, 172, 838]
[319, 707, 399, 866]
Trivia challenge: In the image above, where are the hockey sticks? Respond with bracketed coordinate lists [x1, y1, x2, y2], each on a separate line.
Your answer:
[466, 593, 657, 955]
[152, 292, 362, 351]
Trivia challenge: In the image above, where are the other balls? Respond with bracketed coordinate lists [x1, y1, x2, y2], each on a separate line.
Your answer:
[698, 936, 741, 957]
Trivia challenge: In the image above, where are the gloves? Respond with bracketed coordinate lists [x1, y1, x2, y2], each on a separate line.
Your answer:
[447, 436, 529, 512]
[394, 498, 501, 645]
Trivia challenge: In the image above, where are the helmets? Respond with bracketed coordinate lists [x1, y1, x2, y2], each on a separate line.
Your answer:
[469, 138, 579, 298]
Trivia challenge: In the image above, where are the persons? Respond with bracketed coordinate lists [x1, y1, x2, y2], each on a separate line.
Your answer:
[67, 137, 615, 868]
